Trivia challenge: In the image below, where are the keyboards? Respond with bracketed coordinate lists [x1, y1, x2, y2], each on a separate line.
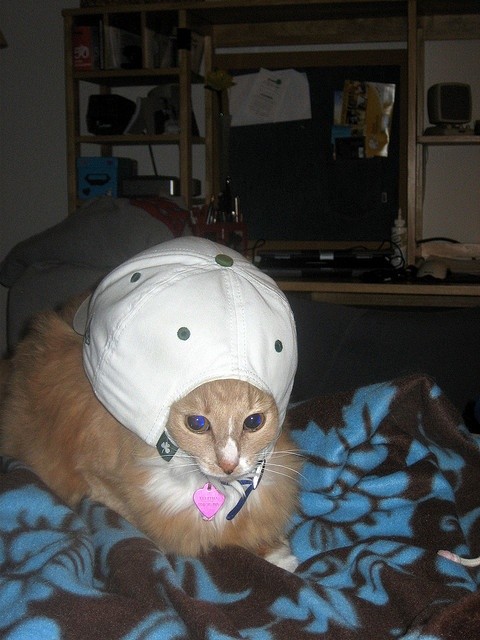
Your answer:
[254, 250, 395, 282]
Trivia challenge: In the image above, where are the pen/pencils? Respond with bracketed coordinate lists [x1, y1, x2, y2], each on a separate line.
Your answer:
[191, 175, 243, 225]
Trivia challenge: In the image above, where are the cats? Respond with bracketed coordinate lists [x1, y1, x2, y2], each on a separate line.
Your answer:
[0, 295, 314, 574]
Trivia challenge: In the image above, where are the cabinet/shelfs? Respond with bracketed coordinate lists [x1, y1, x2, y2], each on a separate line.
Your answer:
[61, 0, 480, 307]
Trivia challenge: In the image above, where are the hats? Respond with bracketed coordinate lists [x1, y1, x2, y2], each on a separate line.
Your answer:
[71, 235, 297, 462]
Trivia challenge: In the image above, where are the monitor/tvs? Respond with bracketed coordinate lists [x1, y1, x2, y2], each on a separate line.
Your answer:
[218, 65, 399, 241]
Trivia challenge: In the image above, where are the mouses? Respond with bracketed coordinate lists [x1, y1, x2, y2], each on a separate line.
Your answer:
[416, 257, 448, 282]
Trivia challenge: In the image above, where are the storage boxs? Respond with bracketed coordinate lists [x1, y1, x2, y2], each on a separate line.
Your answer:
[76, 155, 139, 204]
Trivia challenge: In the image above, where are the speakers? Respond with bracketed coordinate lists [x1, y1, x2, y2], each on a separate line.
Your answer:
[424, 83, 473, 135]
